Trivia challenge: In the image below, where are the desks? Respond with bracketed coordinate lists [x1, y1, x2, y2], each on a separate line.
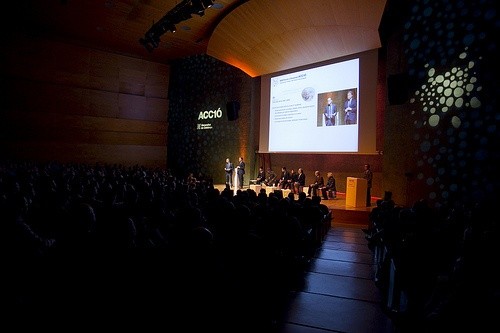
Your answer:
[282, 189, 291, 198]
[250, 184, 261, 197]
[266, 187, 277, 197]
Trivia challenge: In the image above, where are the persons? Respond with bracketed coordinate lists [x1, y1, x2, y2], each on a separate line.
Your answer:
[324, 97, 337, 126]
[362, 190, 500, 333]
[306, 171, 324, 197]
[237, 157, 245, 188]
[224, 158, 234, 188]
[322, 173, 336, 200]
[344, 90, 357, 125]
[363, 165, 373, 206]
[0, 163, 336, 333]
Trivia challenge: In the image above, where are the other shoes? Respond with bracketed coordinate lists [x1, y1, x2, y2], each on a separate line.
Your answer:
[322, 197, 328, 200]
[307, 195, 311, 197]
[361, 229, 371, 235]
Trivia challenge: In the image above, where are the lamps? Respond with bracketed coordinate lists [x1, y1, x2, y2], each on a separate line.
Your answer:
[140, 0, 214, 54]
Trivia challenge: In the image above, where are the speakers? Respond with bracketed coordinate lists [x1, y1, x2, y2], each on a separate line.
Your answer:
[227, 102, 239, 122]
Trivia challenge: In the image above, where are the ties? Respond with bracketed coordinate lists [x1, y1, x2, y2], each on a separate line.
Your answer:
[330, 105, 331, 118]
